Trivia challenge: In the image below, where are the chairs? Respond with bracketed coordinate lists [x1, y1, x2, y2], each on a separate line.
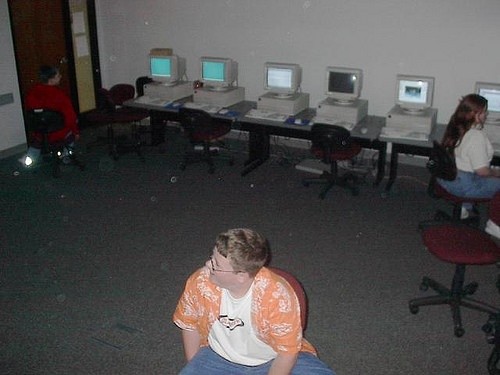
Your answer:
[87, 76, 153, 162]
[426, 140, 491, 228]
[302, 123, 362, 200]
[178, 108, 233, 174]
[27, 108, 85, 179]
[266, 267, 306, 330]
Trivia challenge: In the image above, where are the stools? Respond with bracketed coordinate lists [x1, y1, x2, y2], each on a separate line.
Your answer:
[409, 225, 500, 337]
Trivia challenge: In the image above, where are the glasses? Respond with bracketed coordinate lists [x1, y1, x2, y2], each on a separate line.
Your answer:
[207, 252, 247, 274]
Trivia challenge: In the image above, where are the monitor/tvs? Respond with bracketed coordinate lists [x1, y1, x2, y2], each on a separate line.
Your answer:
[474, 81, 500, 124]
[148, 54, 187, 86]
[262, 62, 302, 99]
[324, 66, 362, 104]
[395, 75, 435, 115]
[199, 57, 238, 91]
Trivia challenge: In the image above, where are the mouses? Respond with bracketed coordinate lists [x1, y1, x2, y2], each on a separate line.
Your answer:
[220, 109, 229, 114]
[174, 102, 182, 106]
[295, 119, 302, 124]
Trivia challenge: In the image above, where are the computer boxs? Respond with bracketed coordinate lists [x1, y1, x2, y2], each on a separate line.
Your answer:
[192, 86, 245, 107]
[143, 81, 195, 102]
[386, 105, 437, 135]
[316, 98, 368, 125]
[257, 92, 309, 116]
[483, 123, 500, 145]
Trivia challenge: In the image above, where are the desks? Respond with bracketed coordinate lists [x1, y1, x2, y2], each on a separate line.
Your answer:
[123, 96, 500, 192]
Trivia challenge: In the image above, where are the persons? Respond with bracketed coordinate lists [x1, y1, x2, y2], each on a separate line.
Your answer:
[173, 227, 335, 375]
[24, 64, 80, 144]
[437, 94, 500, 238]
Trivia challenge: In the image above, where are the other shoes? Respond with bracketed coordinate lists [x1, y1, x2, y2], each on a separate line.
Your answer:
[461, 206, 468, 219]
[485, 218, 500, 238]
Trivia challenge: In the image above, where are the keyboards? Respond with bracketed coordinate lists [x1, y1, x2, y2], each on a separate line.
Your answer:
[379, 128, 429, 141]
[135, 95, 173, 107]
[492, 144, 500, 152]
[246, 109, 289, 122]
[314, 117, 354, 130]
[184, 102, 223, 113]
